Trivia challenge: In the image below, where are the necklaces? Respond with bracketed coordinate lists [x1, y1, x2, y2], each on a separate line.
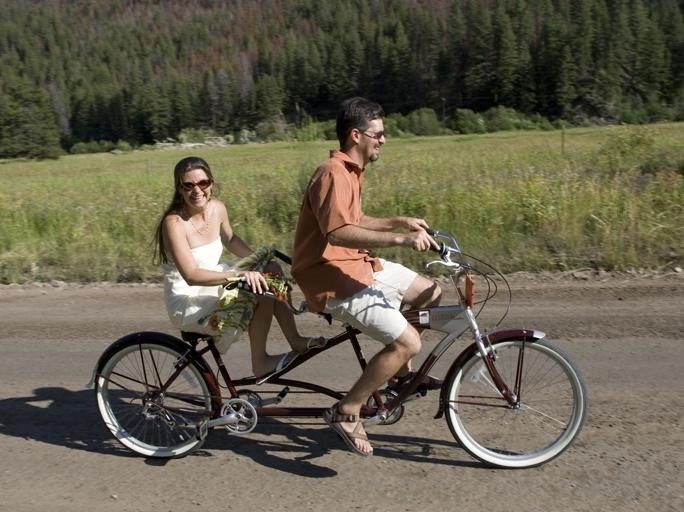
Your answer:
[183, 207, 209, 234]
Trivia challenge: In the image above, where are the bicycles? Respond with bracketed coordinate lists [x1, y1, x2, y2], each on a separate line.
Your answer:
[87, 219, 590, 472]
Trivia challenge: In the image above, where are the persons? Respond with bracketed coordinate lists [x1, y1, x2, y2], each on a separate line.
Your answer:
[290, 97, 443, 458]
[148, 157, 329, 385]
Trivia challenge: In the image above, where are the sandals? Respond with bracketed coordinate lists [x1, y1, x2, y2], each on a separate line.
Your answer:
[386, 371, 443, 394]
[323, 401, 373, 458]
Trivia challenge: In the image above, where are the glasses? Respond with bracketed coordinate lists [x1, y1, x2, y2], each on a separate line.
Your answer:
[180, 180, 211, 191]
[358, 130, 385, 138]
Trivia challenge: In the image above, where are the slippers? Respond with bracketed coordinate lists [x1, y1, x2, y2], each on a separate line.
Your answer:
[256, 337, 328, 385]
